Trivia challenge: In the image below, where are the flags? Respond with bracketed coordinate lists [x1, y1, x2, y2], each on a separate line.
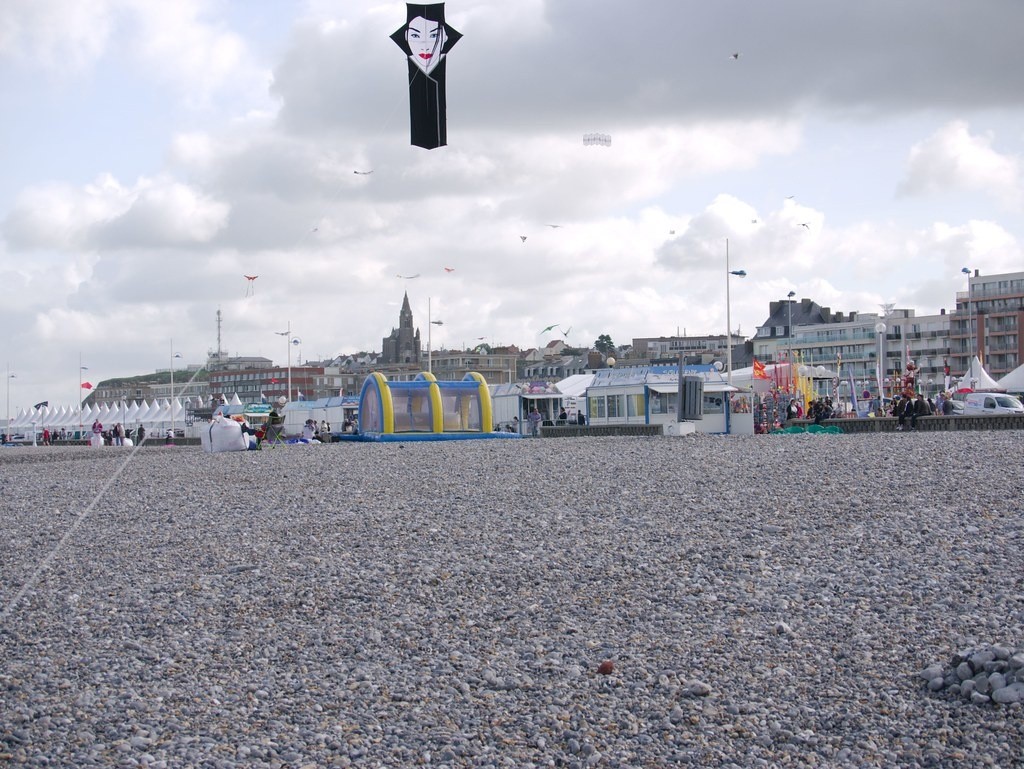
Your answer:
[82, 382, 92, 389]
[753, 360, 770, 379]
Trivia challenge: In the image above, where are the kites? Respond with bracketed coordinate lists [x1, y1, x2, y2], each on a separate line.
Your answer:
[34, 400, 48, 410]
[431, 321, 444, 326]
[244, 275, 258, 297]
[520, 236, 527, 242]
[444, 267, 455, 273]
[390, 2, 464, 150]
[582, 132, 612, 147]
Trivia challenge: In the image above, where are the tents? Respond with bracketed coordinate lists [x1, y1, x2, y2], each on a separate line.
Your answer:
[4, 393, 242, 437]
[944, 356, 1002, 389]
[721, 364, 839, 401]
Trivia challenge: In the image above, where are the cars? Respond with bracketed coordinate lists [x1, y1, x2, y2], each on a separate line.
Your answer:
[903, 398, 964, 415]
[166, 428, 185, 438]
[964, 391, 1024, 416]
[849, 397, 895, 418]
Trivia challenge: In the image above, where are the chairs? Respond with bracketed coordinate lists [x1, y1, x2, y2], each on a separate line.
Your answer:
[256, 415, 287, 450]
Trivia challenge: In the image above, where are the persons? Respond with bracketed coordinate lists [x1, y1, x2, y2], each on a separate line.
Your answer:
[92, 419, 103, 448]
[495, 406, 586, 437]
[788, 392, 954, 433]
[104, 422, 126, 446]
[302, 418, 360, 444]
[138, 424, 146, 446]
[42, 427, 66, 446]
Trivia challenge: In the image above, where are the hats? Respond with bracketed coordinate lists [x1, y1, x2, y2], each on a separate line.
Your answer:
[896, 396, 900, 400]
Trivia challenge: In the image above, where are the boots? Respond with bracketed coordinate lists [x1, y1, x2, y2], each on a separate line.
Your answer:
[899, 424, 904, 430]
[896, 424, 901, 429]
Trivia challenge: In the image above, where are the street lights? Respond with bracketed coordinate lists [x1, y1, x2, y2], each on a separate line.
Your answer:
[6, 366, 16, 441]
[875, 321, 887, 410]
[962, 267, 975, 392]
[798, 364, 826, 402]
[884, 376, 900, 400]
[78, 351, 89, 439]
[840, 380, 848, 415]
[787, 291, 796, 398]
[952, 376, 963, 391]
[428, 296, 443, 373]
[168, 340, 182, 438]
[724, 238, 747, 436]
[917, 379, 933, 399]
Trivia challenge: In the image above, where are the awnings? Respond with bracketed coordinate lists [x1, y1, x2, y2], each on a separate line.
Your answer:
[522, 394, 573, 399]
